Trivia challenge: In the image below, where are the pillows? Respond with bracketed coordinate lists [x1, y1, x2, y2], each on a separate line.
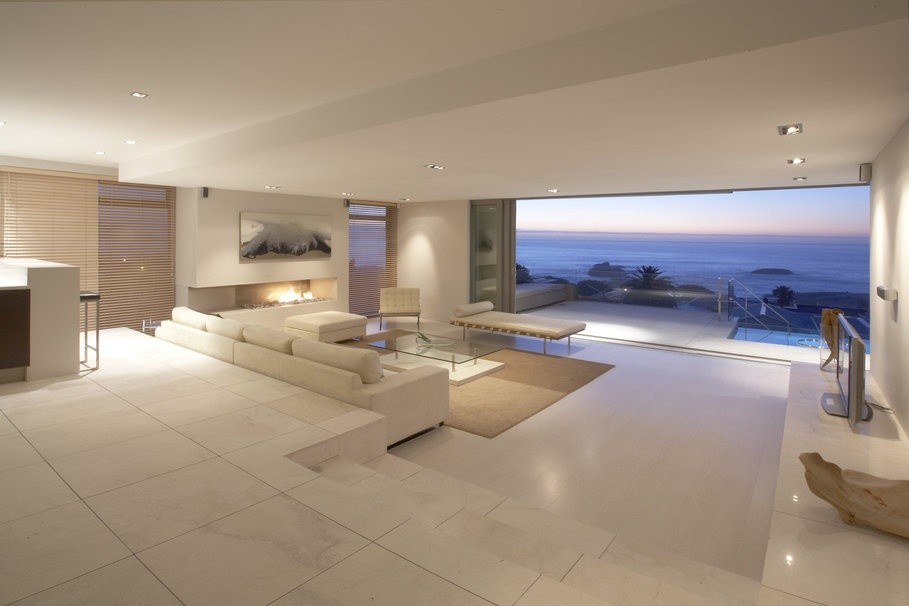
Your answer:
[454, 301, 493, 316]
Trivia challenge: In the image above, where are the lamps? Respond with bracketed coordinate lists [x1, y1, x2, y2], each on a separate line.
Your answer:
[201, 187, 208, 197]
[344, 199, 350, 208]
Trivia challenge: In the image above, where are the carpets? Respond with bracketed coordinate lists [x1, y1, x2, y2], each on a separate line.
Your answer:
[334, 329, 616, 439]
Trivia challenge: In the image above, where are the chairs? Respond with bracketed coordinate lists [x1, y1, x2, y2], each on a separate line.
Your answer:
[379, 287, 421, 331]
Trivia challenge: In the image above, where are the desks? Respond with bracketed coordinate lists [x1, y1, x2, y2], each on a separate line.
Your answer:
[143, 319, 160, 333]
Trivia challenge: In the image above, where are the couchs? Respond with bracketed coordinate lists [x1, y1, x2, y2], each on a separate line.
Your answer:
[154, 306, 450, 448]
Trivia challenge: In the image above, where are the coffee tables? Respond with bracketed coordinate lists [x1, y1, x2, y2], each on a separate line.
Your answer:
[365, 332, 506, 387]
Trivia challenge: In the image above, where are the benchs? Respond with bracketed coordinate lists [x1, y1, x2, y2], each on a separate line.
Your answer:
[284, 310, 367, 343]
[450, 311, 586, 355]
[479, 269, 577, 313]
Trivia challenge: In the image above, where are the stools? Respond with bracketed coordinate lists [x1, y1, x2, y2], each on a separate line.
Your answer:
[80, 290, 102, 371]
